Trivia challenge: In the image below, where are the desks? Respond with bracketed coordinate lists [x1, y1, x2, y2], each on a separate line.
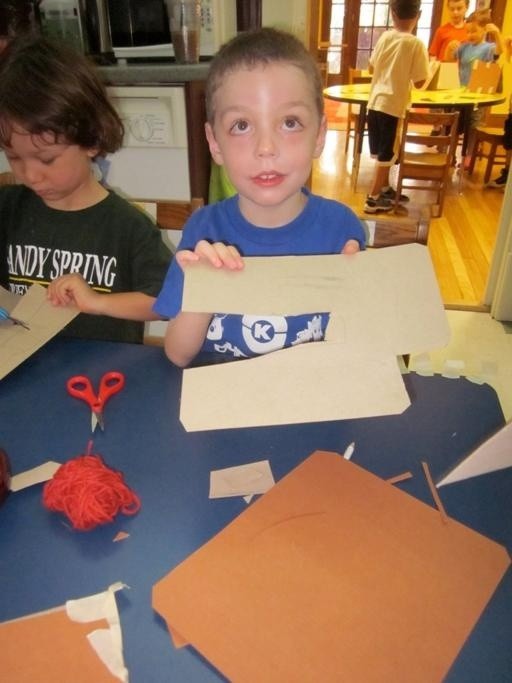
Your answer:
[0, 339, 512, 680]
[322, 83, 506, 193]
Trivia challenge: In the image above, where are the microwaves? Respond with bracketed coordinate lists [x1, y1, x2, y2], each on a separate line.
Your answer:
[105, 0, 240, 62]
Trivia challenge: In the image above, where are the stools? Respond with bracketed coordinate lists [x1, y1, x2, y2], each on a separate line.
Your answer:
[466, 128, 506, 182]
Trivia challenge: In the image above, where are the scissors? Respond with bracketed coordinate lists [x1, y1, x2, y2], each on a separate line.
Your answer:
[0, 308, 30, 330]
[67, 372, 124, 432]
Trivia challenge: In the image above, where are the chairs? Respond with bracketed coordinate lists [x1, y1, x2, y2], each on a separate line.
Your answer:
[393, 110, 460, 218]
[343, 65, 374, 157]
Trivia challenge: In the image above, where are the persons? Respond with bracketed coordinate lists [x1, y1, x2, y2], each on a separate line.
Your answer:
[486, 35, 512, 189]
[426, 0, 469, 148]
[0, 38, 176, 345]
[362, 0, 429, 214]
[150, 27, 366, 369]
[442, 11, 504, 168]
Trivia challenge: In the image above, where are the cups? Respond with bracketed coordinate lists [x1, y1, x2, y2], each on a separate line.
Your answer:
[169, 15, 201, 64]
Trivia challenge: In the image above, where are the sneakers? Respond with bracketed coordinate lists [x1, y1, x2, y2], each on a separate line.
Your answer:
[449, 156, 457, 176]
[380, 187, 409, 206]
[427, 126, 442, 147]
[363, 193, 393, 212]
[489, 168, 508, 187]
